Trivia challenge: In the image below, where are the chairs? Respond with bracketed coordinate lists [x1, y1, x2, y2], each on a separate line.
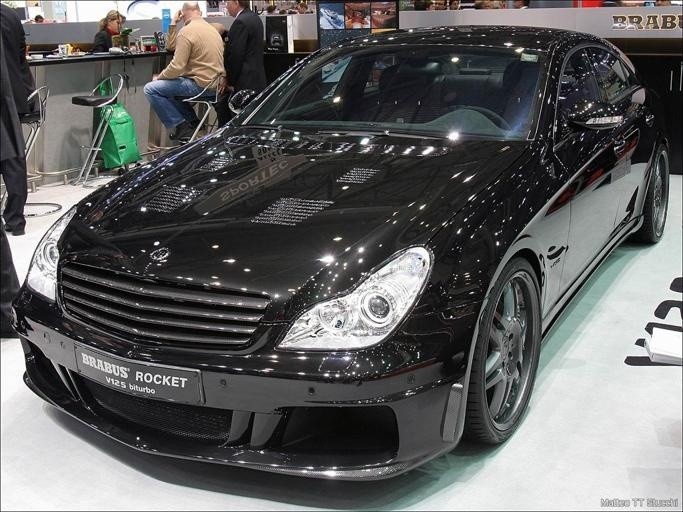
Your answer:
[351, 60, 544, 144]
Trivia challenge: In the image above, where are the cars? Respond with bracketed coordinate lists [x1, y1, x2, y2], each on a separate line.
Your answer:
[9, 21, 671, 488]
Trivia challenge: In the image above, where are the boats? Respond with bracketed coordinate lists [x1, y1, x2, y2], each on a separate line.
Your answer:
[320, 8, 344, 29]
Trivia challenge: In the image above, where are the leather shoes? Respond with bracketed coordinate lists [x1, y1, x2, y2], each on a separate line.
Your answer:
[169, 121, 194, 140]
[190, 118, 206, 131]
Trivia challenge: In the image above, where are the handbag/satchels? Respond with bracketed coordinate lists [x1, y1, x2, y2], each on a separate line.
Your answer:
[99, 78, 143, 169]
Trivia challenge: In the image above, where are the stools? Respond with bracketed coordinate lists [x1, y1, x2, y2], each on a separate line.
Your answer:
[0, 86, 64, 220]
[155, 72, 227, 166]
[69, 73, 129, 188]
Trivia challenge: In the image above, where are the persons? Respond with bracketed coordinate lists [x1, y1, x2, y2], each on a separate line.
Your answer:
[0, 28, 24, 338]
[141, 1, 225, 143]
[209, 0, 267, 131]
[34, 14, 43, 23]
[412, 0, 530, 10]
[265, 2, 314, 15]
[0, 2, 33, 237]
[615, 0, 643, 8]
[164, 9, 186, 52]
[653, 0, 670, 6]
[91, 10, 127, 52]
